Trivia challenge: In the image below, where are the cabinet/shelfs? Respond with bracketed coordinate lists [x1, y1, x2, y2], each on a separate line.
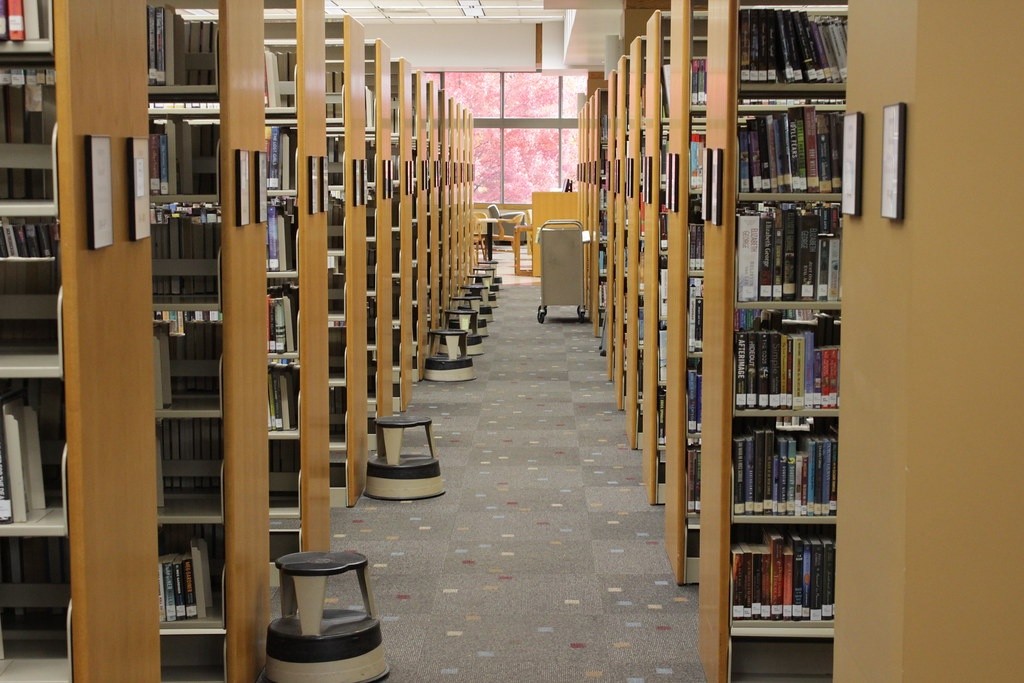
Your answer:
[0, 0, 854, 683]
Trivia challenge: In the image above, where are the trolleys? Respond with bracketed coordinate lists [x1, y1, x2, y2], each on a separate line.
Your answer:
[536, 220, 586, 325]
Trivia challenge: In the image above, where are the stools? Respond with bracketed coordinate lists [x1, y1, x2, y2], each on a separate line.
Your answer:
[424, 329, 475, 381]
[458, 284, 488, 306]
[449, 296, 487, 329]
[470, 261, 503, 283]
[472, 267, 502, 283]
[439, 309, 485, 355]
[265, 548, 391, 682]
[362, 415, 446, 502]
[467, 274, 496, 301]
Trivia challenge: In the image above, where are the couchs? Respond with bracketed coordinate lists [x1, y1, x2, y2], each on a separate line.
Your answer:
[488, 204, 528, 246]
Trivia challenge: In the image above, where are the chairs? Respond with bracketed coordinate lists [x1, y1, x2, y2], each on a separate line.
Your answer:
[485, 214, 523, 266]
[473, 212, 488, 261]
[525, 209, 533, 253]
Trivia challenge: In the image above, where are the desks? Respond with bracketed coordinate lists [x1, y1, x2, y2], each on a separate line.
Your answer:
[477, 218, 498, 264]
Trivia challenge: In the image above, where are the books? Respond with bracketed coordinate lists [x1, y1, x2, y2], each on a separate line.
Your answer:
[598, 9, 848, 622]
[0, 0, 343, 623]
